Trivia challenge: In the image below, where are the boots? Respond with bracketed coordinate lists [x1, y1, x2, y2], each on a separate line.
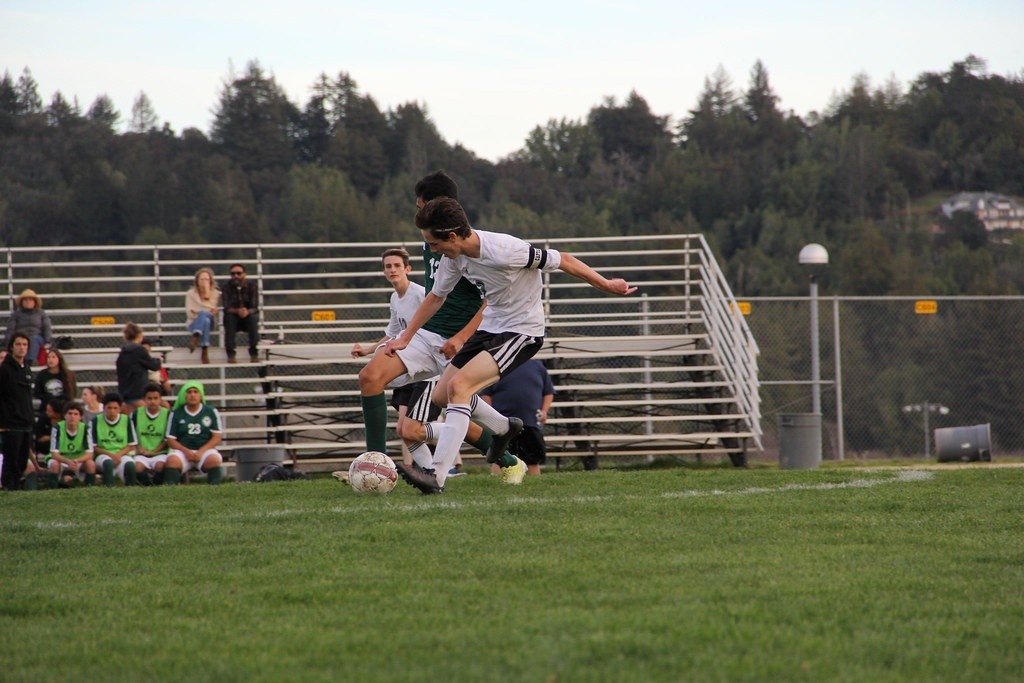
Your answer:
[188, 336, 200, 352]
[201, 349, 210, 364]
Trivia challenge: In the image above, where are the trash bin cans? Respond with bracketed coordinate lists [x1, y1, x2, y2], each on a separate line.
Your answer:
[231, 446, 287, 481]
[932, 422, 992, 464]
[776, 412, 824, 469]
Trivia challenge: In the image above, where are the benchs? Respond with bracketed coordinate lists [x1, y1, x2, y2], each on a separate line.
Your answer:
[30, 328, 765, 478]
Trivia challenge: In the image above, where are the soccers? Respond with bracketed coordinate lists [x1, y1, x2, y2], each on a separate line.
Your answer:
[349, 450, 399, 494]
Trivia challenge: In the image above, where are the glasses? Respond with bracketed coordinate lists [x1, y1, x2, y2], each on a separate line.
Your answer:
[229, 271, 244, 277]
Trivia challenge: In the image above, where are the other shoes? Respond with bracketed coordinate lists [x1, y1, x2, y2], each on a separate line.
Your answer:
[227, 352, 237, 364]
[251, 352, 258, 363]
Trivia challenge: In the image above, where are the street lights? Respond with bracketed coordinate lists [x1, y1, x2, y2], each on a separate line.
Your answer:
[795, 244, 829, 413]
[901, 403, 949, 459]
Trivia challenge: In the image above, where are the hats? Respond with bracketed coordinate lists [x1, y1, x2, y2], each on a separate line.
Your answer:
[16, 290, 42, 309]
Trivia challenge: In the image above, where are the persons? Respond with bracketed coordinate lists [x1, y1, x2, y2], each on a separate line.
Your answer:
[164, 380, 222, 487]
[477, 357, 554, 477]
[0, 288, 54, 368]
[220, 264, 261, 363]
[88, 392, 139, 487]
[0, 348, 9, 365]
[32, 350, 76, 424]
[347, 168, 638, 491]
[80, 384, 105, 423]
[130, 383, 172, 485]
[0, 333, 33, 491]
[117, 323, 171, 413]
[186, 267, 221, 362]
[0, 399, 96, 487]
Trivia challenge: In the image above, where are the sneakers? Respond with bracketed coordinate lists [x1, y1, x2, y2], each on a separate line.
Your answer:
[500, 456, 529, 484]
[486, 417, 525, 463]
[332, 470, 351, 484]
[394, 461, 444, 494]
